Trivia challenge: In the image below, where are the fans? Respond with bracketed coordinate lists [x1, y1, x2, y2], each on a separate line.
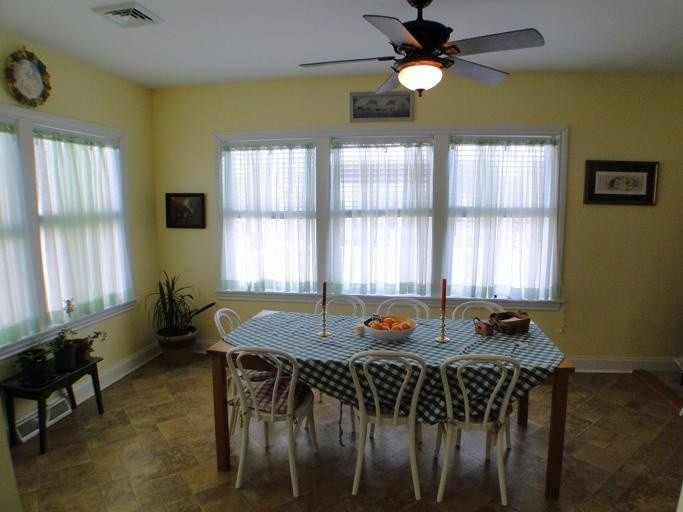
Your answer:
[295, 1, 546, 96]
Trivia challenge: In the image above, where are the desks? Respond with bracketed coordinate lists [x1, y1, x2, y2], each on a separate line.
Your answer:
[0, 354, 103, 454]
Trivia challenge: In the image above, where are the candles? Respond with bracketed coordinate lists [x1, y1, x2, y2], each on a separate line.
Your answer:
[439, 278, 446, 311]
[321, 281, 326, 306]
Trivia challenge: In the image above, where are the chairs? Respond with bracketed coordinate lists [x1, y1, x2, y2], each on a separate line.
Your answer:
[314, 292, 368, 404]
[451, 300, 515, 452]
[350, 297, 432, 437]
[348, 350, 425, 500]
[213, 307, 310, 445]
[225, 344, 317, 498]
[433, 354, 520, 506]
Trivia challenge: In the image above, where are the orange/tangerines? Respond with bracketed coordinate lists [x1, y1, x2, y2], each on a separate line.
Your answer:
[368, 318, 410, 330]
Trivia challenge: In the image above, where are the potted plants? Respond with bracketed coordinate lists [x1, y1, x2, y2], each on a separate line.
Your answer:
[49, 328, 108, 369]
[135, 270, 216, 366]
[8, 342, 55, 384]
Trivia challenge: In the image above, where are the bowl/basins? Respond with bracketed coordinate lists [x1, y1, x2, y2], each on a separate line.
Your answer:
[363, 316, 418, 341]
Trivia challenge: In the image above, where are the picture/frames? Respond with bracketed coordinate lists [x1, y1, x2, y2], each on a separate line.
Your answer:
[164, 192, 205, 229]
[348, 90, 413, 123]
[581, 159, 659, 207]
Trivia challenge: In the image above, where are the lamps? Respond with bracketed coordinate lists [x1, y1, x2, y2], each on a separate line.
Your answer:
[392, 52, 447, 98]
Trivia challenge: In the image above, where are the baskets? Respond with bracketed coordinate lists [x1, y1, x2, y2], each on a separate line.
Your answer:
[489, 309, 531, 335]
